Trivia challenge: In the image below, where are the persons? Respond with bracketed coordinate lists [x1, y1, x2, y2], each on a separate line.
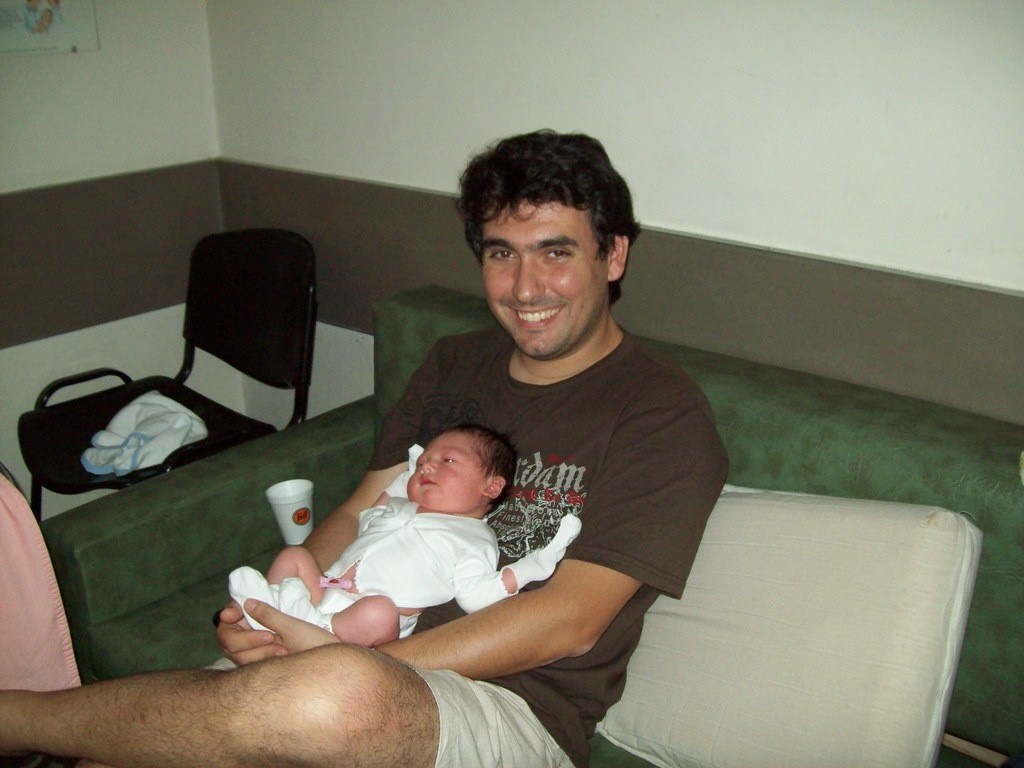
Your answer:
[228, 422, 582, 649]
[0, 128, 731, 767]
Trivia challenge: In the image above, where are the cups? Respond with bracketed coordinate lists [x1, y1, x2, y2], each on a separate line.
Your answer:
[265, 479, 313, 545]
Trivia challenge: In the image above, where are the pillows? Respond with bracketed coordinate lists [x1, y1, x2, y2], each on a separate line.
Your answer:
[595, 484, 982, 768]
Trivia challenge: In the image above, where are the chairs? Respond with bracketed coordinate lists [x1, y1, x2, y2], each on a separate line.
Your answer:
[17, 227, 319, 522]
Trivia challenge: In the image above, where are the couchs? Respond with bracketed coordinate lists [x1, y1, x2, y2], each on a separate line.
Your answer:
[38, 281, 1024, 768]
